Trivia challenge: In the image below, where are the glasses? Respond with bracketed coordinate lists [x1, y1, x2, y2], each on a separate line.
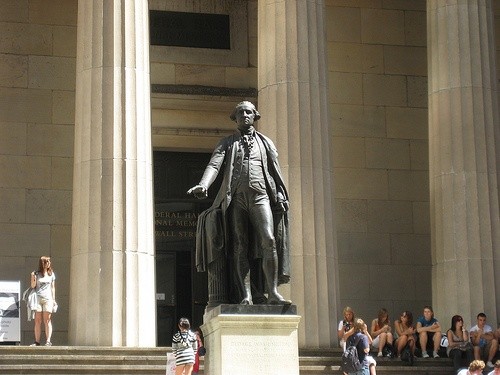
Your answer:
[401, 315, 406, 318]
[457, 319, 463, 322]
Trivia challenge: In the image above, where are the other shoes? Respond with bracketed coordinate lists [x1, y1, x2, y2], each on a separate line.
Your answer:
[378, 353, 382, 357]
[44, 341, 52, 346]
[387, 352, 392, 358]
[29, 342, 40, 346]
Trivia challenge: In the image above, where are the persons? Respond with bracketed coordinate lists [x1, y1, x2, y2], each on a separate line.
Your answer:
[416, 306, 442, 358]
[393, 310, 418, 359]
[172, 318, 203, 375]
[338, 306, 377, 375]
[370, 307, 394, 357]
[187, 101, 293, 305]
[447, 313, 500, 375]
[28, 256, 56, 346]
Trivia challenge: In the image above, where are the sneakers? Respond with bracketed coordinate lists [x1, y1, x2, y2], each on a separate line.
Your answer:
[433, 353, 439, 358]
[423, 353, 429, 358]
[487, 362, 494, 367]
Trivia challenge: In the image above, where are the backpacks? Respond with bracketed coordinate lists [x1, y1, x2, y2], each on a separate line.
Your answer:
[341, 334, 365, 375]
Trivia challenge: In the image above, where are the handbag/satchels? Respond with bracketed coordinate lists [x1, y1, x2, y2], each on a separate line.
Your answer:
[383, 342, 393, 356]
[401, 341, 411, 361]
